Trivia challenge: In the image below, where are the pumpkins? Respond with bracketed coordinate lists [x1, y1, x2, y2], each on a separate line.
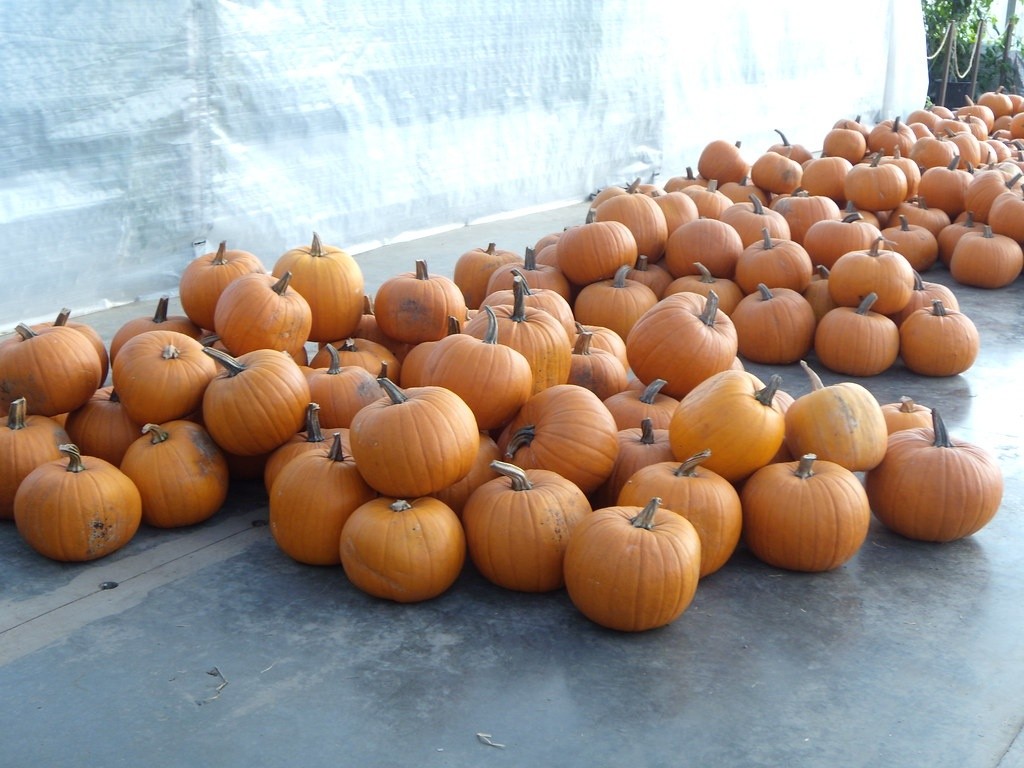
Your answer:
[0, 87, 1024, 633]
